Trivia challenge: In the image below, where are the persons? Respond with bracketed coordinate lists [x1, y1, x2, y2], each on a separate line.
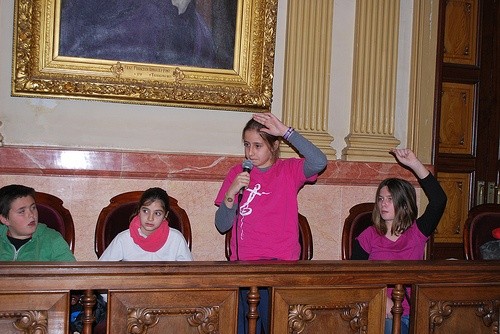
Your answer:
[351, 149, 447, 334]
[214, 112, 328, 334]
[0, 184, 77, 262]
[98, 187, 194, 303]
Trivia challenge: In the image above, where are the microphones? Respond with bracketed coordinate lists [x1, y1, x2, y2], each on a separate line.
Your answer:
[238, 159, 253, 201]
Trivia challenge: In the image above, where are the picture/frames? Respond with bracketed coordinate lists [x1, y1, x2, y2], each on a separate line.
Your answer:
[443, 0, 481, 68]
[437, 77, 480, 158]
[11, 1, 279, 113]
[433, 166, 478, 248]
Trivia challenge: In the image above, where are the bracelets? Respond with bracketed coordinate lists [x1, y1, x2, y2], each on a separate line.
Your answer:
[283, 127, 294, 139]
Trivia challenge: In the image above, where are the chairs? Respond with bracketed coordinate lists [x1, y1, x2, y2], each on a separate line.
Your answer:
[95, 191, 192, 259]
[34, 191, 75, 253]
[225, 212, 313, 260]
[463, 204, 500, 260]
[342, 202, 375, 260]
